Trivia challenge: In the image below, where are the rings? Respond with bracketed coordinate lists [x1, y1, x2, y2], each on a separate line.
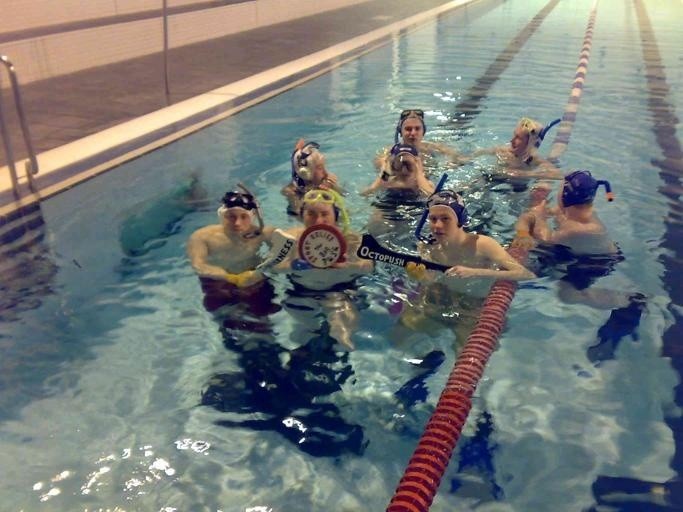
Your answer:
[448, 267, 454, 272]
[450, 273, 459, 278]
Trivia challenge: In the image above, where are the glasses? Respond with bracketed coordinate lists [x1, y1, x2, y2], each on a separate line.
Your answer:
[519, 116, 536, 134]
[303, 189, 335, 206]
[427, 190, 458, 205]
[390, 157, 415, 174]
[400, 109, 423, 119]
[298, 141, 319, 160]
[222, 191, 254, 209]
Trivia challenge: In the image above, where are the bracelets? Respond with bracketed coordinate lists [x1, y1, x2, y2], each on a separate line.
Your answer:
[418, 182, 429, 188]
[517, 228, 530, 239]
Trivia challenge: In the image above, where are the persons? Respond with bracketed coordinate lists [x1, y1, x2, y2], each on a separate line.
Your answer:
[513, 169, 645, 311]
[393, 190, 532, 362]
[445, 118, 563, 215]
[270, 191, 374, 349]
[362, 144, 435, 240]
[188, 189, 289, 344]
[372, 107, 466, 180]
[284, 140, 348, 218]
